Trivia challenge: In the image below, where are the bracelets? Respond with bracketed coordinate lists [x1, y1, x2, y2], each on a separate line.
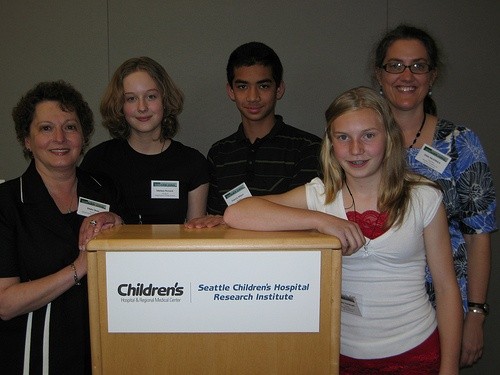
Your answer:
[468, 307, 487, 317]
[70, 261, 80, 286]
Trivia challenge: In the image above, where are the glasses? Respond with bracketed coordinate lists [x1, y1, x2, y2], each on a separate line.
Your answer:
[382, 62, 432, 74]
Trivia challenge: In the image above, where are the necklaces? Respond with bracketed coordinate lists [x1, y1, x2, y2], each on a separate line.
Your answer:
[44, 182, 76, 214]
[409, 111, 428, 149]
[344, 177, 384, 253]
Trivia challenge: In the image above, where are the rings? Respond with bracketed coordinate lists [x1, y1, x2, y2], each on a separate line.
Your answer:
[90, 220, 97, 225]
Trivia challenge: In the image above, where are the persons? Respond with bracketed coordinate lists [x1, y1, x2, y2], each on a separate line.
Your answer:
[78, 56, 213, 225]
[374, 25, 500, 369]
[0, 80, 125, 375]
[224, 87, 462, 375]
[182, 42, 324, 229]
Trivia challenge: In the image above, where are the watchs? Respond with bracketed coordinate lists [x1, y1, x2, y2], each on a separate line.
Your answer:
[466, 300, 491, 313]
